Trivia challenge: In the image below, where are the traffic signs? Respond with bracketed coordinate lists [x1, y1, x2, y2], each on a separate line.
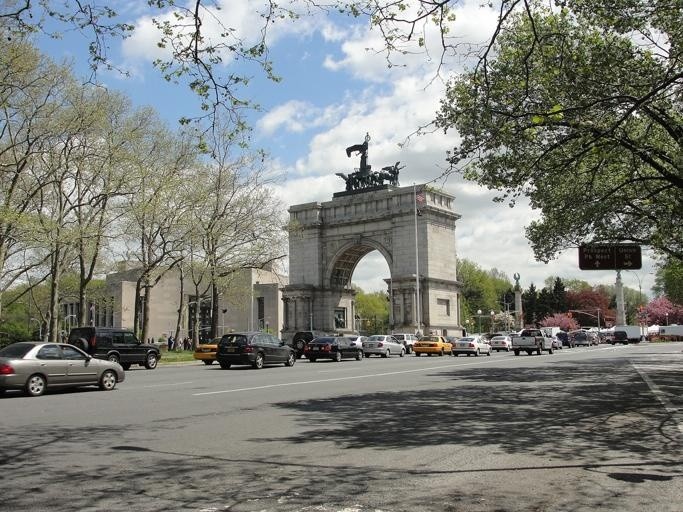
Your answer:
[578, 242, 641, 270]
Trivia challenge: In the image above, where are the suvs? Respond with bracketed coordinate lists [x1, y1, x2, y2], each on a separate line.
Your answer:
[68, 327, 161, 369]
[293, 330, 329, 358]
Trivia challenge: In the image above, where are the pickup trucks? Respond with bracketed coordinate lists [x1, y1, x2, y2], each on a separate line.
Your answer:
[512, 327, 555, 355]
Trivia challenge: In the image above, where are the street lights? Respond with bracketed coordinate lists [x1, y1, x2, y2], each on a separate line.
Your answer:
[495, 299, 513, 332]
[63, 315, 75, 338]
[466, 310, 495, 334]
[567, 309, 600, 330]
[626, 269, 653, 324]
[257, 316, 270, 330]
[29, 317, 43, 339]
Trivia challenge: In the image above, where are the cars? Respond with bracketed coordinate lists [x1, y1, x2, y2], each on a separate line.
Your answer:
[412, 334, 512, 356]
[216, 331, 296, 369]
[194, 337, 222, 364]
[0, 342, 125, 396]
[305, 334, 418, 362]
[539, 325, 628, 349]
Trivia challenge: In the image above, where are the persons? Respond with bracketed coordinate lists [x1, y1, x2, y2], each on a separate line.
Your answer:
[200, 336, 214, 344]
[152, 338, 154, 343]
[355, 313, 360, 319]
[148, 338, 150, 343]
[168, 336, 192, 351]
